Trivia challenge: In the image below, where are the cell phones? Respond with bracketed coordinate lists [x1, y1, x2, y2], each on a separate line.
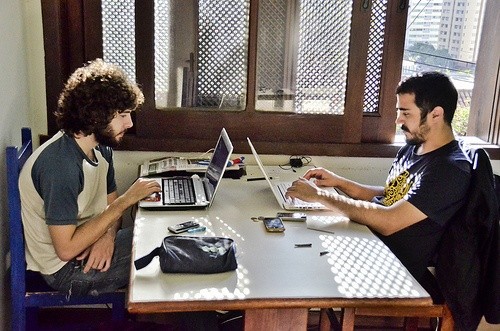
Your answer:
[263, 216, 286, 232]
[277, 211, 307, 220]
[168, 220, 199, 233]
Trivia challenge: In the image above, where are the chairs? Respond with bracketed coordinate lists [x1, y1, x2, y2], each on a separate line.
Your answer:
[317, 135, 500, 331]
[5, 126, 128, 331]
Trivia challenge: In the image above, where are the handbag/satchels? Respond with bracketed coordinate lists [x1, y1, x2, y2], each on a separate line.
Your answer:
[134, 234, 237, 273]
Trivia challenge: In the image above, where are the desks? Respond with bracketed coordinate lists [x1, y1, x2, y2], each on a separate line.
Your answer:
[127, 165, 435, 331]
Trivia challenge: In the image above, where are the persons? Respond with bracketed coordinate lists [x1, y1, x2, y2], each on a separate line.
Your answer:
[18, 60, 162, 294]
[285, 72, 471, 280]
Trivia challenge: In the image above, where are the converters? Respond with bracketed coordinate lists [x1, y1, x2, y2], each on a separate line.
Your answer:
[289, 158, 303, 168]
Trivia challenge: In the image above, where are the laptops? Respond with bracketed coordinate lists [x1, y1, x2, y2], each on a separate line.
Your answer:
[246, 136, 343, 211]
[139, 127, 233, 210]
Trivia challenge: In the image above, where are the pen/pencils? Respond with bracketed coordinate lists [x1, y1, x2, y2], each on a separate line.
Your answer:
[246, 177, 278, 181]
[258, 216, 306, 222]
[307, 227, 334, 233]
